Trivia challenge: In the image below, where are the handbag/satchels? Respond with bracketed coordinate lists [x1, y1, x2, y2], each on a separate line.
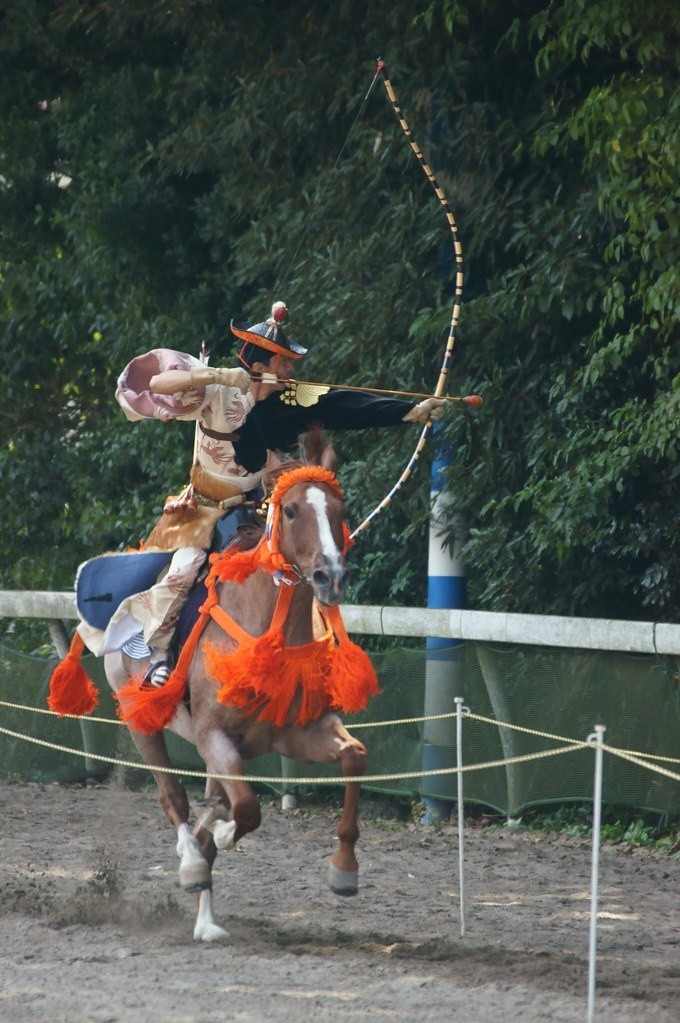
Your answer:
[143, 494, 229, 554]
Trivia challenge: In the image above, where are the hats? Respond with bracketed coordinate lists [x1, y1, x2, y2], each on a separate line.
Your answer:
[227, 300, 310, 359]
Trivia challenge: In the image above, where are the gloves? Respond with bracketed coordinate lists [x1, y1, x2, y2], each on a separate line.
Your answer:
[401, 398, 451, 424]
[188, 363, 252, 394]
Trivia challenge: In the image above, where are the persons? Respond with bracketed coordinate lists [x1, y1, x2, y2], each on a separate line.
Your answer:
[75, 299, 451, 689]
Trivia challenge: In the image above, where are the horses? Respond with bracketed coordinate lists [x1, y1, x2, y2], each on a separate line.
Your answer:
[102, 423, 368, 943]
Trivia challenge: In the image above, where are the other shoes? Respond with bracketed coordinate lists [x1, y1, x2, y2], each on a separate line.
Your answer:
[146, 659, 173, 692]
